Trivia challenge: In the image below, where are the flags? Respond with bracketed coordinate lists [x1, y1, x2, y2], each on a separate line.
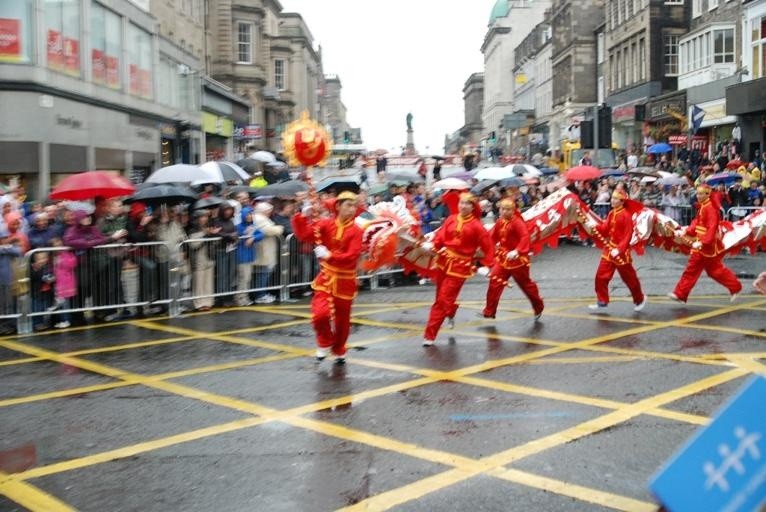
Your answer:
[689, 105, 706, 140]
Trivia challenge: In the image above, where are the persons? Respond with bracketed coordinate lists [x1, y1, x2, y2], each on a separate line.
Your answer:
[0, 144, 766, 331]
[293, 190, 364, 363]
[419, 190, 498, 347]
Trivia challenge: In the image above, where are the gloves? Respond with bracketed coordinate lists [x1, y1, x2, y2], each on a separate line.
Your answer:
[610, 248, 621, 259]
[477, 266, 488, 278]
[585, 220, 598, 232]
[313, 242, 332, 262]
[300, 200, 319, 217]
[422, 241, 435, 252]
[506, 250, 519, 260]
[694, 242, 703, 249]
[674, 230, 687, 238]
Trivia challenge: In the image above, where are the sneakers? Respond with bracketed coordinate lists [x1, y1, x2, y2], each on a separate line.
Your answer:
[729, 284, 742, 299]
[315, 340, 328, 358]
[475, 310, 498, 320]
[589, 302, 610, 310]
[633, 295, 647, 310]
[667, 292, 687, 305]
[448, 317, 455, 331]
[174, 289, 313, 313]
[333, 354, 345, 364]
[532, 311, 543, 321]
[0, 305, 164, 335]
[422, 337, 433, 345]
